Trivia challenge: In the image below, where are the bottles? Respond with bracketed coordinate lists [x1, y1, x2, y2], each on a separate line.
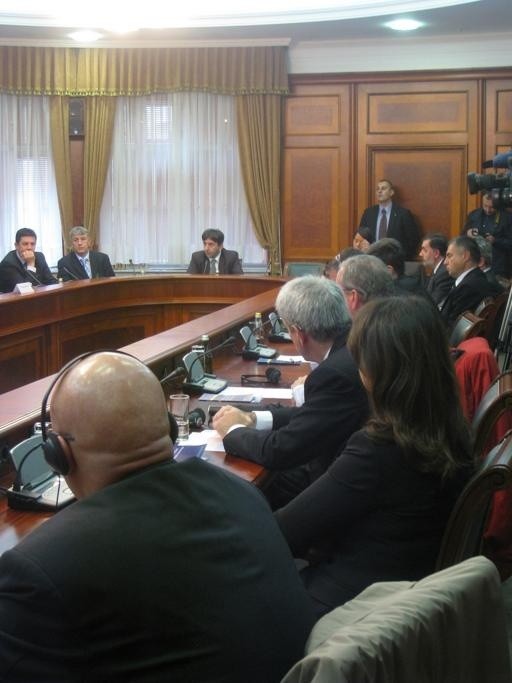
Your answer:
[57, 276, 62, 282]
[198, 334, 214, 373]
[255, 312, 265, 339]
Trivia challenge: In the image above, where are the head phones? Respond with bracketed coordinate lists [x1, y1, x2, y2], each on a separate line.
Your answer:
[241, 366, 280, 388]
[39, 346, 181, 477]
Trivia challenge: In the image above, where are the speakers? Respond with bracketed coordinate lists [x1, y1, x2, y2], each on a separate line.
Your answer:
[67, 95, 86, 139]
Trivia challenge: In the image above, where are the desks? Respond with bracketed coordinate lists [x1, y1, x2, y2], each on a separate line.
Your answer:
[0, 268, 318, 561]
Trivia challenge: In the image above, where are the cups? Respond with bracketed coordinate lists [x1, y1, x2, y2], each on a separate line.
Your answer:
[248, 320, 260, 342]
[168, 393, 191, 439]
[191, 344, 205, 373]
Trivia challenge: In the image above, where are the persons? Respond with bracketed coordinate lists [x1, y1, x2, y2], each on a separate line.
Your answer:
[324, 225, 495, 329]
[213, 274, 370, 512]
[57, 225, 114, 281]
[186, 229, 243, 275]
[459, 193, 512, 281]
[358, 179, 419, 262]
[0, 349, 319, 683]
[257, 294, 482, 617]
[0, 227, 58, 294]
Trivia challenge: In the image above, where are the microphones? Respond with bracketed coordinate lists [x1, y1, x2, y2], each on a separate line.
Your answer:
[7, 442, 43, 511]
[241, 315, 282, 360]
[186, 333, 239, 395]
[269, 317, 291, 344]
[201, 256, 210, 275]
[22, 263, 49, 287]
[58, 260, 80, 282]
[127, 258, 137, 274]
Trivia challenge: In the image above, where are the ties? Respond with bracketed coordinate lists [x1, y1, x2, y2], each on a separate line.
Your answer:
[81, 259, 91, 278]
[210, 260, 216, 274]
[377, 209, 387, 240]
